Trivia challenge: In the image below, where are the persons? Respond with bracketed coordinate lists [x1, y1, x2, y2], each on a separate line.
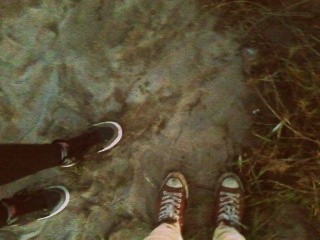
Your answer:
[140, 171, 248, 240]
[0, 120, 123, 229]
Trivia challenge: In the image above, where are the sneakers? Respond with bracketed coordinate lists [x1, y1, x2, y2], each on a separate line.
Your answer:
[5, 183, 69, 228]
[154, 171, 188, 233]
[53, 121, 122, 167]
[212, 173, 245, 232]
